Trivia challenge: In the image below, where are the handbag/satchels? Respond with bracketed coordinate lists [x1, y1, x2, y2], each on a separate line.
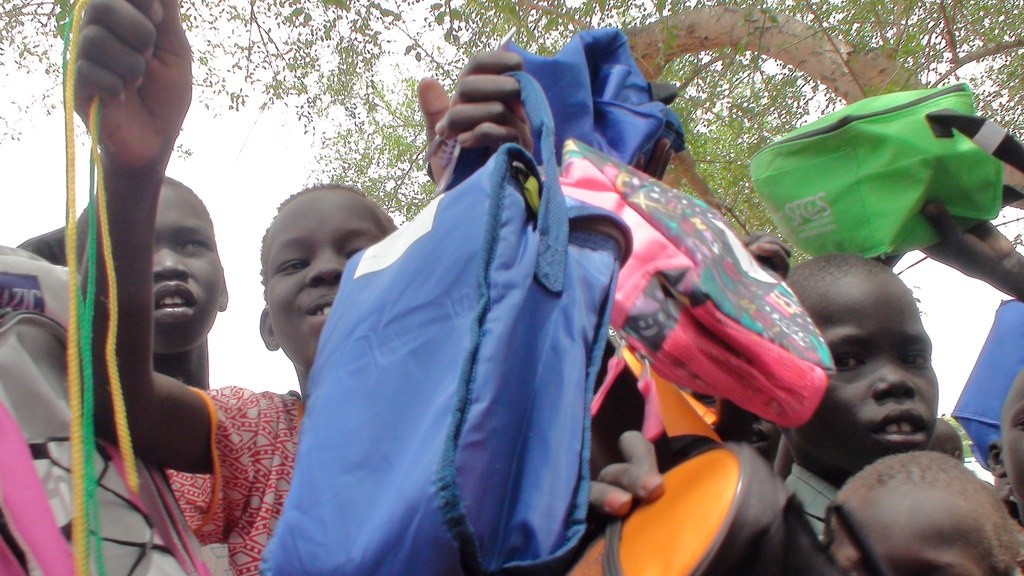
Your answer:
[951, 295, 1024, 471]
[0, 310, 213, 576]
[746, 84, 1024, 268]
[260, 28, 837, 576]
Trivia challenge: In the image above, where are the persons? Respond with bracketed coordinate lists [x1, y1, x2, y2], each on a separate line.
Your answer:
[0, 0, 1024, 575]
